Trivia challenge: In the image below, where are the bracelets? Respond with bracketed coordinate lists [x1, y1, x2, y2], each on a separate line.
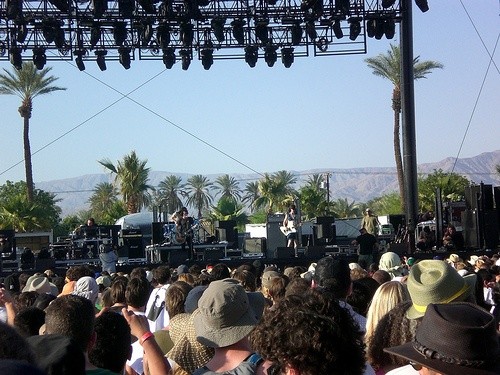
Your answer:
[139, 331, 153, 346]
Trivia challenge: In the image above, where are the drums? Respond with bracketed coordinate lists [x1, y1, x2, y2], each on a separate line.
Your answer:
[164, 224, 176, 237]
[171, 231, 186, 245]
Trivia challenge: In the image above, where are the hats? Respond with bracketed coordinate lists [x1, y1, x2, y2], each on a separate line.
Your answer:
[349, 263, 361, 270]
[289, 204, 295, 209]
[177, 265, 190, 275]
[252, 260, 318, 288]
[100, 244, 106, 250]
[379, 252, 500, 375]
[3, 270, 128, 302]
[365, 208, 372, 212]
[26, 335, 86, 375]
[314, 256, 350, 288]
[146, 270, 154, 282]
[161, 278, 265, 374]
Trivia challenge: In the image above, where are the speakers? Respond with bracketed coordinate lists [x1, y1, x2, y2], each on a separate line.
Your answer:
[461, 184, 500, 250]
[266, 213, 295, 259]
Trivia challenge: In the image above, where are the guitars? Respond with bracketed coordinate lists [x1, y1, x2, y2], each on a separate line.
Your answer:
[280, 223, 303, 236]
[174, 223, 199, 243]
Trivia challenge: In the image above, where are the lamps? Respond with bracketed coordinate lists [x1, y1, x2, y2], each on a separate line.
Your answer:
[0, 0, 430, 72]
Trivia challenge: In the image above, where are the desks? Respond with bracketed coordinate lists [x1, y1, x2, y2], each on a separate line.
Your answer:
[145, 243, 228, 264]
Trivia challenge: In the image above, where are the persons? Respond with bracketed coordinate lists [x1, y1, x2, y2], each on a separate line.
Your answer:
[350, 228, 377, 269]
[175, 210, 196, 260]
[417, 226, 435, 252]
[99, 244, 119, 273]
[442, 225, 458, 252]
[360, 207, 381, 237]
[80, 217, 99, 238]
[171, 207, 187, 223]
[0, 248, 500, 375]
[282, 204, 302, 258]
